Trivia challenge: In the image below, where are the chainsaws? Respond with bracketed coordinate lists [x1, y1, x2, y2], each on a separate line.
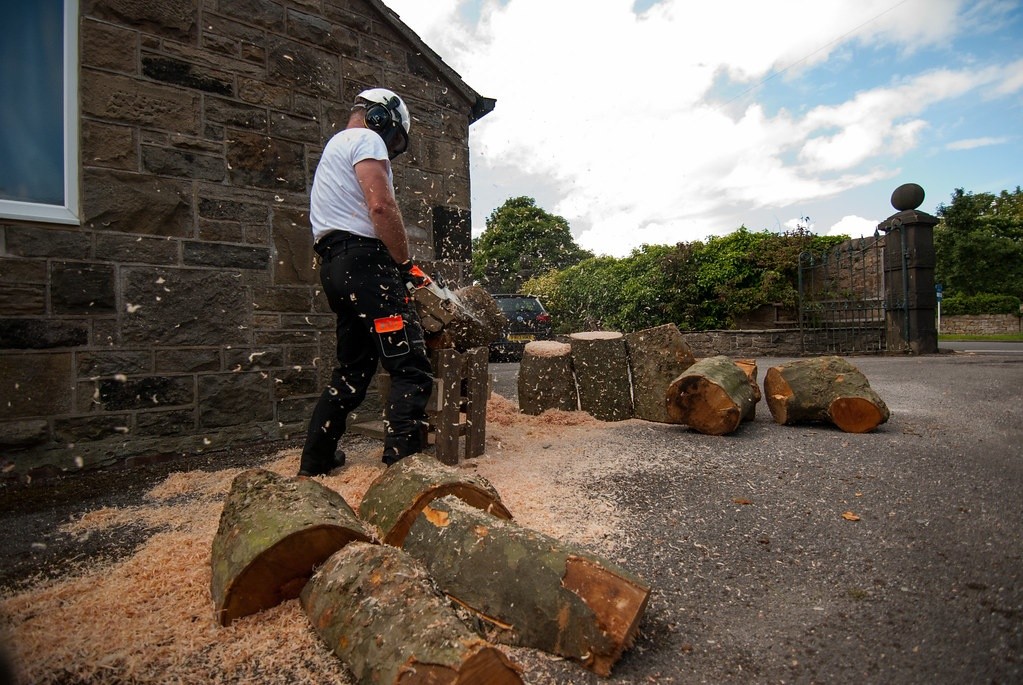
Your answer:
[408, 270, 489, 338]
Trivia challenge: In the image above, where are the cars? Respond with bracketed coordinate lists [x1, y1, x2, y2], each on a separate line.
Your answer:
[488, 292, 555, 360]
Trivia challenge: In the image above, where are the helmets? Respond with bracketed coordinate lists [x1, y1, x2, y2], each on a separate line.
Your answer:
[354, 88, 410, 135]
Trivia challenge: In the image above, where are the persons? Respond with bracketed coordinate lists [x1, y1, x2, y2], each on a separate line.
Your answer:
[297, 88, 433, 476]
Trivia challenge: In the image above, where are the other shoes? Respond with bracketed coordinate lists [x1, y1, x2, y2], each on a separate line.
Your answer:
[298, 450, 345, 477]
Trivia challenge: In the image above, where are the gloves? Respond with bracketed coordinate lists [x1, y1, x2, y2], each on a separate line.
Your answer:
[397, 257, 432, 294]
